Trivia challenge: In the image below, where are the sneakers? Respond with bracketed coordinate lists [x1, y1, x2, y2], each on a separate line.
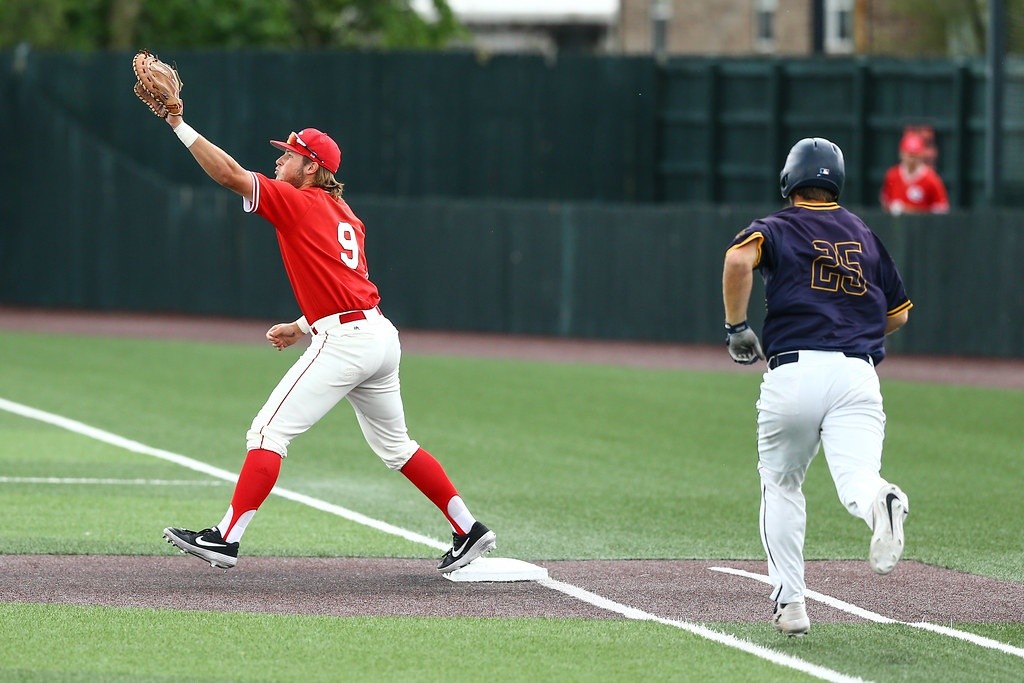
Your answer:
[436, 522, 498, 576]
[869, 483, 904, 575]
[771, 604, 810, 639]
[162, 526, 240, 570]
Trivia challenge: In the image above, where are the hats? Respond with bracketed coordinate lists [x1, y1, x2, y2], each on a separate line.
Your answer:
[899, 129, 926, 156]
[270, 128, 342, 175]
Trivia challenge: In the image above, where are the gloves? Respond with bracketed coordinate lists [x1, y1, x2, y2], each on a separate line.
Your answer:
[724, 322, 765, 365]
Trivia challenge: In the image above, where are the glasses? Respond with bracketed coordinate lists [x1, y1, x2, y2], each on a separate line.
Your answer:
[286, 132, 324, 164]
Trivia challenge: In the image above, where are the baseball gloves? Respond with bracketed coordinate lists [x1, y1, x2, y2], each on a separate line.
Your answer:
[132, 51, 184, 118]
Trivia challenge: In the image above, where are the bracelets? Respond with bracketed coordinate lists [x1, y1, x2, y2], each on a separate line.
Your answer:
[174, 122, 202, 148]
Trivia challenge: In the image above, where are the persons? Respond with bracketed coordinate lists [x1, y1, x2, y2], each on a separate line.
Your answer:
[165, 114, 497, 574]
[881, 131, 947, 217]
[723, 138, 914, 637]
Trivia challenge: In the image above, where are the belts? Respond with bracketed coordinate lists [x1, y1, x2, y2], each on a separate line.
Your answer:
[770, 352, 870, 370]
[312, 306, 382, 336]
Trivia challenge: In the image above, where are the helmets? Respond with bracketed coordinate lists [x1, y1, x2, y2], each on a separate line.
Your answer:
[780, 137, 845, 199]
